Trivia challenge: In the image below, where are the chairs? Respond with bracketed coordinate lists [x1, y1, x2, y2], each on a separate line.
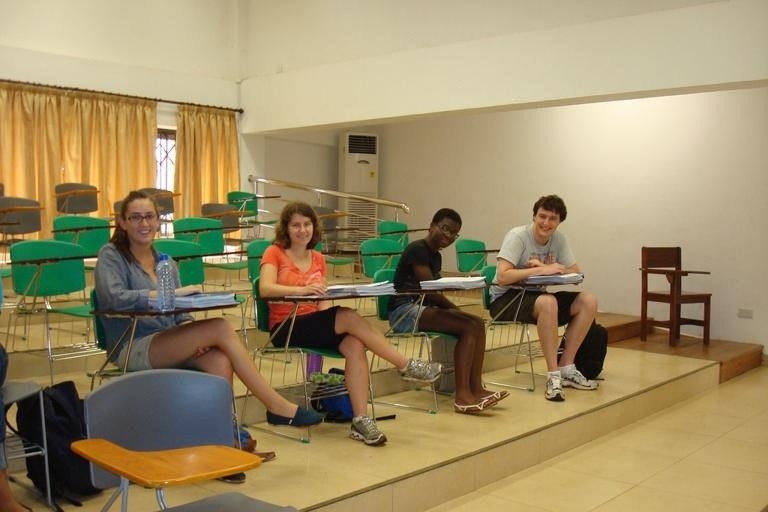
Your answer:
[0, 176, 569, 511]
[638, 245, 712, 351]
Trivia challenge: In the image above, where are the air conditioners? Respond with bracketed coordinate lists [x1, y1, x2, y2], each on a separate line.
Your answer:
[337, 132, 380, 252]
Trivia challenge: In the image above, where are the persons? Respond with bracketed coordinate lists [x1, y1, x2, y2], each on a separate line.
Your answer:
[388, 206, 511, 416]
[256, 201, 445, 447]
[93, 189, 325, 484]
[487, 192, 599, 402]
[0, 342, 32, 512]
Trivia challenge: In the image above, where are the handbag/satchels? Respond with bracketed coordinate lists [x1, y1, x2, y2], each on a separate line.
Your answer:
[313, 369, 364, 423]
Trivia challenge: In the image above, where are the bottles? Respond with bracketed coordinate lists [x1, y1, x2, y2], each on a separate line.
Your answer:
[156, 254, 176, 310]
[306, 351, 322, 381]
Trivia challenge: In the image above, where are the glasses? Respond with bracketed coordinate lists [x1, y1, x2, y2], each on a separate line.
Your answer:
[433, 223, 459, 240]
[125, 213, 157, 224]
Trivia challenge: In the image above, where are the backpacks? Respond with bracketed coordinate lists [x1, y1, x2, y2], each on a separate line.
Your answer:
[558, 318, 610, 381]
[19, 378, 108, 505]
[429, 336, 465, 395]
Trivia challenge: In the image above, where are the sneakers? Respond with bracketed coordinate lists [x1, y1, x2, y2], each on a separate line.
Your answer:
[398, 357, 444, 385]
[348, 415, 388, 447]
[265, 400, 324, 427]
[544, 364, 602, 402]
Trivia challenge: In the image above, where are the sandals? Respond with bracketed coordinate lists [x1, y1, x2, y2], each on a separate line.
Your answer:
[453, 388, 511, 415]
